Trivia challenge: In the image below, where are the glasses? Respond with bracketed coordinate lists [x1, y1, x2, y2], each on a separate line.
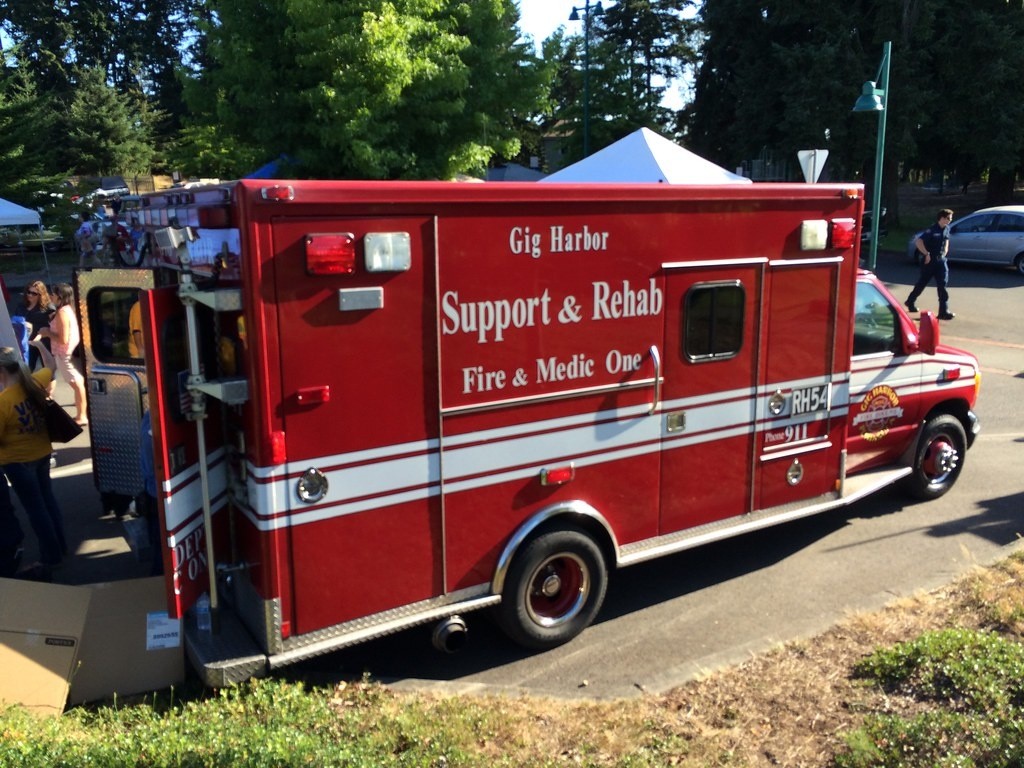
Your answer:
[26, 289, 37, 296]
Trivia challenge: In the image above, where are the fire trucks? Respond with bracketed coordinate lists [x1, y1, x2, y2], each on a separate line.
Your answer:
[71, 129, 978, 689]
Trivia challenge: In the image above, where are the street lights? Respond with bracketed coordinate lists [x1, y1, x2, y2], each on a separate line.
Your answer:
[570, 0, 605, 158]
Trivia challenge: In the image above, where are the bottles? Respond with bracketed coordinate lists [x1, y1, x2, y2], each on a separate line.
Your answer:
[196, 591, 211, 629]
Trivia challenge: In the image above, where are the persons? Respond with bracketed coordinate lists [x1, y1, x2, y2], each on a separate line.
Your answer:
[904, 209, 955, 320]
[0, 334, 68, 566]
[16, 280, 89, 426]
[75, 193, 130, 267]
[127, 301, 145, 366]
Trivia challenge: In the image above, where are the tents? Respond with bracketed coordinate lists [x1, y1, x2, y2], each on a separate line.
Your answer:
[402, 127, 752, 185]
[243, 153, 307, 179]
[0, 198, 50, 278]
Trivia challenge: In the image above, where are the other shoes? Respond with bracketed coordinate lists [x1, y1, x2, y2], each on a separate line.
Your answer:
[938, 311, 953, 320]
[904, 299, 918, 313]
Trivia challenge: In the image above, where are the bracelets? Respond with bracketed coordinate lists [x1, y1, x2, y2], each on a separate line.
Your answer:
[925, 252, 930, 255]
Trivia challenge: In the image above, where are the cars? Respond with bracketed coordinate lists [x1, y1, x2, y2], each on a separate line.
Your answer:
[860, 205, 890, 244]
[44, 242, 76, 253]
[0, 244, 28, 253]
[908, 204, 1024, 277]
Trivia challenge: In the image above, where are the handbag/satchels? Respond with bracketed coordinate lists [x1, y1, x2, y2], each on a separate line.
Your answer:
[43, 399, 83, 444]
